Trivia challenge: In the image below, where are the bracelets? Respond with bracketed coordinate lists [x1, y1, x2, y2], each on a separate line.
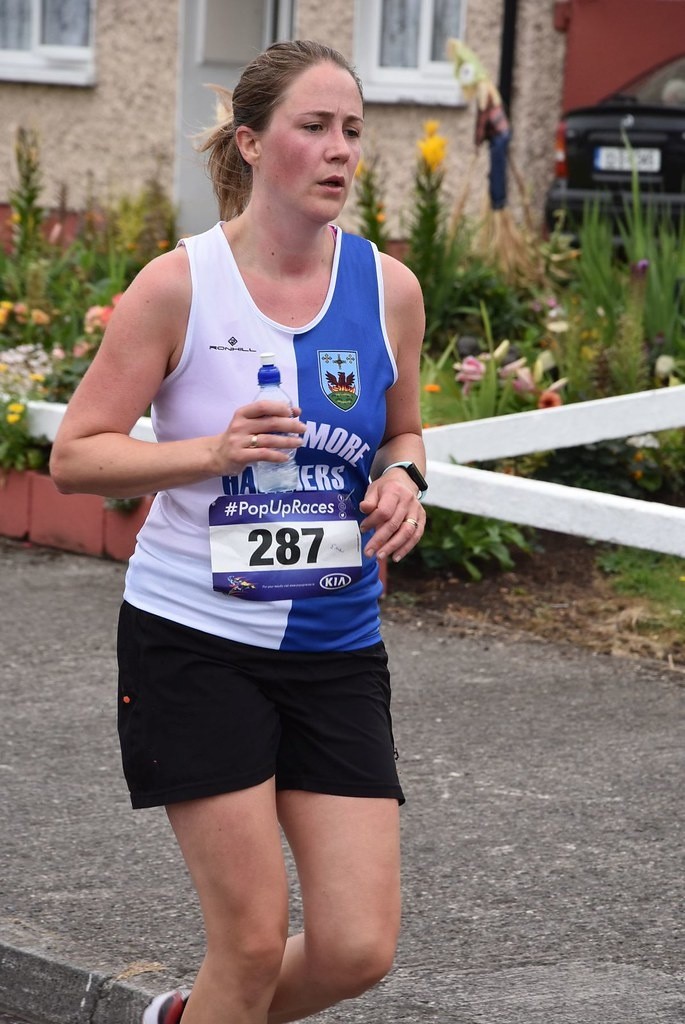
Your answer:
[382, 460, 428, 502]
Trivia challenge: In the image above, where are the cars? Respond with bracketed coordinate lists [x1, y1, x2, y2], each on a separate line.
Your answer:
[545, 57, 685, 263]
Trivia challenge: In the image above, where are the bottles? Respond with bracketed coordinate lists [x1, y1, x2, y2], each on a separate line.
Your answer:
[251, 364, 298, 493]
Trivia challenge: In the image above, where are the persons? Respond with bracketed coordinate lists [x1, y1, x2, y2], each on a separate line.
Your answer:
[49, 42, 430, 1024]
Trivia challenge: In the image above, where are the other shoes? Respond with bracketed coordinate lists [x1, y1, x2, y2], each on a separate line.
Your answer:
[144, 989, 192, 1024]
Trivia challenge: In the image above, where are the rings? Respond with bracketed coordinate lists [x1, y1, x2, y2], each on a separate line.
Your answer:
[403, 518, 419, 529]
[249, 435, 258, 448]
